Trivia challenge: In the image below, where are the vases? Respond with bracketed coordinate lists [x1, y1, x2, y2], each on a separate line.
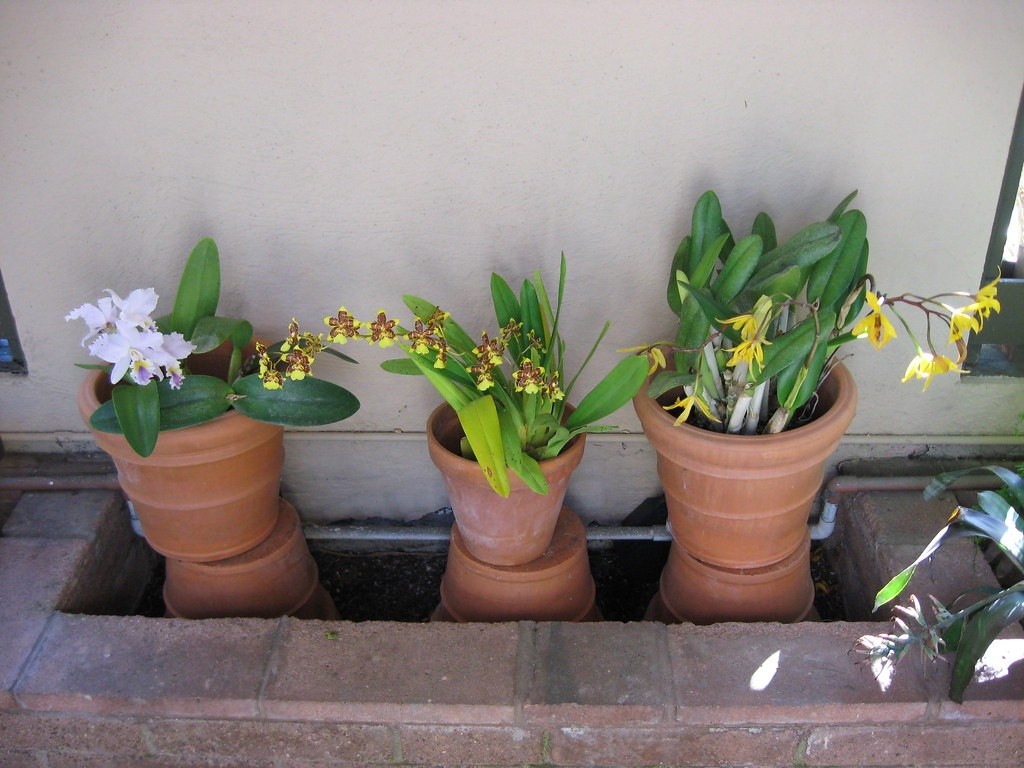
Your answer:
[79, 337, 335, 620]
[426, 390, 608, 623]
[631, 338, 859, 624]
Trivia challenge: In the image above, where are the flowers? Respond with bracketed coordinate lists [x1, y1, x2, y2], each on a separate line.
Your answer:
[615, 191, 1002, 434]
[65, 238, 361, 458]
[254, 252, 650, 498]
